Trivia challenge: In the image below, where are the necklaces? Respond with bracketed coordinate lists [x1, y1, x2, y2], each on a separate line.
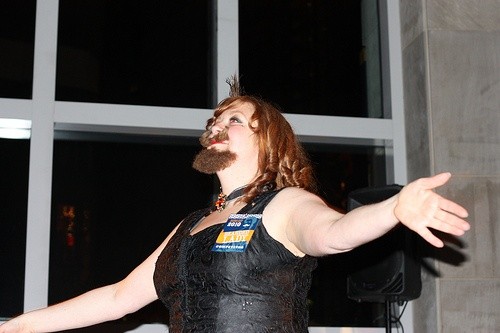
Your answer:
[214, 187, 242, 213]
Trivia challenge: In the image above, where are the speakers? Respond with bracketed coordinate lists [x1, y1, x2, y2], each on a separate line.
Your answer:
[347, 185, 422, 303]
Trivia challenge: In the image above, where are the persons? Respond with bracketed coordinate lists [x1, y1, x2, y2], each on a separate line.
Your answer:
[0, 72, 470, 333]
